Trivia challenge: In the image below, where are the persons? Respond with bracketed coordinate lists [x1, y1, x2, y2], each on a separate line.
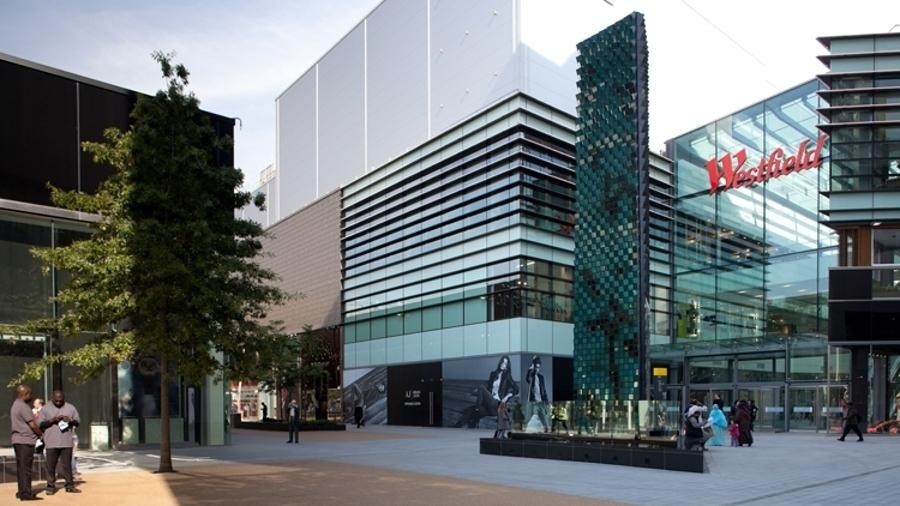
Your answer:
[526, 358, 550, 432]
[38, 390, 81, 496]
[71, 427, 80, 476]
[286, 400, 301, 444]
[10, 384, 45, 502]
[354, 404, 363, 428]
[839, 398, 848, 437]
[494, 392, 512, 439]
[449, 355, 519, 430]
[261, 402, 267, 421]
[837, 402, 863, 442]
[550, 398, 761, 451]
[31, 397, 45, 453]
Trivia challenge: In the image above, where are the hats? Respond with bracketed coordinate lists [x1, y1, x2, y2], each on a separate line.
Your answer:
[687, 404, 708, 418]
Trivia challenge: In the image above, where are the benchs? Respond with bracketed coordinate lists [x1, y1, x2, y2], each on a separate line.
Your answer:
[0, 446, 59, 483]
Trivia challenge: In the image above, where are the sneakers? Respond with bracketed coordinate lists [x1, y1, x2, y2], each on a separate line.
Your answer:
[46, 485, 56, 496]
[66, 487, 82, 493]
[15, 490, 43, 501]
